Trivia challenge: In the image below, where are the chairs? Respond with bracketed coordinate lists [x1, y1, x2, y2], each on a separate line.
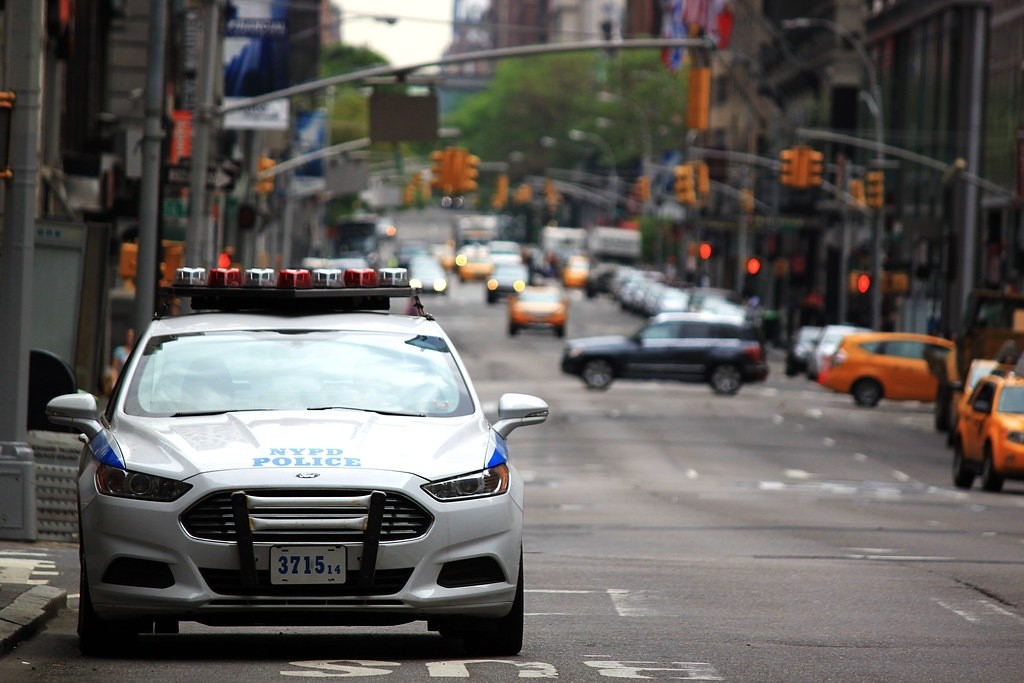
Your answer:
[178, 356, 235, 411]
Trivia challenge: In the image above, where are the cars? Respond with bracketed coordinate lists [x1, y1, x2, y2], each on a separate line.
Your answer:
[953, 360, 1024, 491]
[454, 240, 568, 334]
[397, 239, 450, 294]
[583, 263, 764, 325]
[564, 255, 588, 285]
[45, 306, 551, 654]
[793, 324, 872, 379]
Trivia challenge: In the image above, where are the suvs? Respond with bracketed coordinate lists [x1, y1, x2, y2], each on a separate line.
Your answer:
[817, 332, 956, 406]
[562, 312, 769, 397]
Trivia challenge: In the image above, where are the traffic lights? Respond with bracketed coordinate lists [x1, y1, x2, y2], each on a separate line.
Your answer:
[780, 145, 823, 187]
[431, 146, 479, 195]
[257, 157, 276, 194]
[745, 255, 761, 275]
[866, 171, 885, 205]
[694, 241, 712, 260]
[671, 163, 696, 204]
[851, 270, 872, 295]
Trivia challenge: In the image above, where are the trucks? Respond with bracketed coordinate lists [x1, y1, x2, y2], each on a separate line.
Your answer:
[334, 224, 378, 260]
[540, 223, 642, 297]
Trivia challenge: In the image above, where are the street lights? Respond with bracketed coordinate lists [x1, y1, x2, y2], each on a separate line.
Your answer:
[784, 17, 886, 324]
